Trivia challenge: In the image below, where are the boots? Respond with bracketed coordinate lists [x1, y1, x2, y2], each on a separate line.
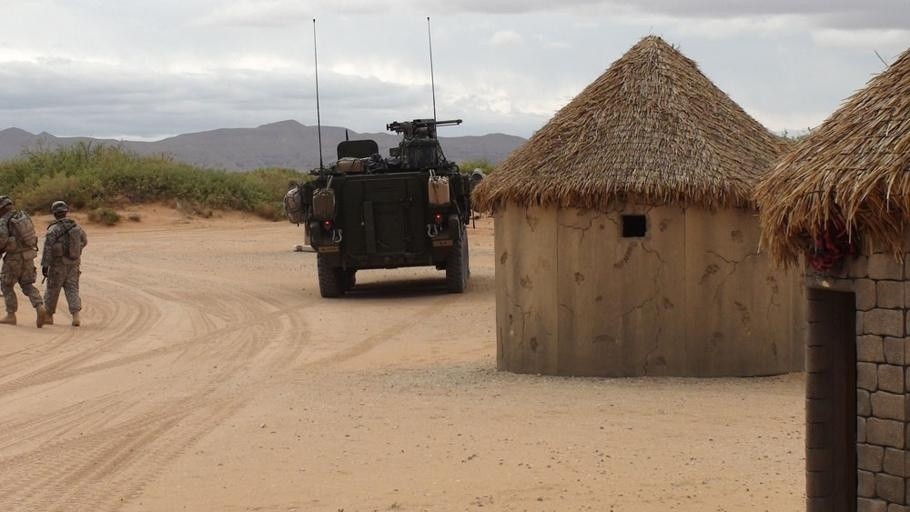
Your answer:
[35, 304, 52, 327]
[0, 312, 16, 324]
[72, 311, 80, 325]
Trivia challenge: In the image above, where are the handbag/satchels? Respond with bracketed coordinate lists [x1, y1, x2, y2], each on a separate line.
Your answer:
[365, 153, 387, 173]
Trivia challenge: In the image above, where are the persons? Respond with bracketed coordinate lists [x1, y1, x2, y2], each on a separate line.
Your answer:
[41, 200, 87, 327]
[0, 195, 46, 328]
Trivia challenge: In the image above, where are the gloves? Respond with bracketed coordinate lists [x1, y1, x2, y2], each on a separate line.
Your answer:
[42, 267, 48, 277]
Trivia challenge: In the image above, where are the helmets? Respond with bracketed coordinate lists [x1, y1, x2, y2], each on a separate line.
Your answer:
[0, 195, 13, 209]
[52, 201, 68, 213]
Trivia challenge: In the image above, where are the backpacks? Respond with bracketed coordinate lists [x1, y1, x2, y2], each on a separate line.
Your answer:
[468, 168, 487, 213]
[338, 157, 363, 173]
[313, 189, 335, 220]
[283, 187, 304, 223]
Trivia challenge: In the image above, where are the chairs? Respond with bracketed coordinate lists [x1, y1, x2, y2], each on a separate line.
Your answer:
[338, 140, 379, 160]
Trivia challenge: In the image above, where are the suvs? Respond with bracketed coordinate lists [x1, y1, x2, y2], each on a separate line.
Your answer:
[295, 118, 483, 297]
